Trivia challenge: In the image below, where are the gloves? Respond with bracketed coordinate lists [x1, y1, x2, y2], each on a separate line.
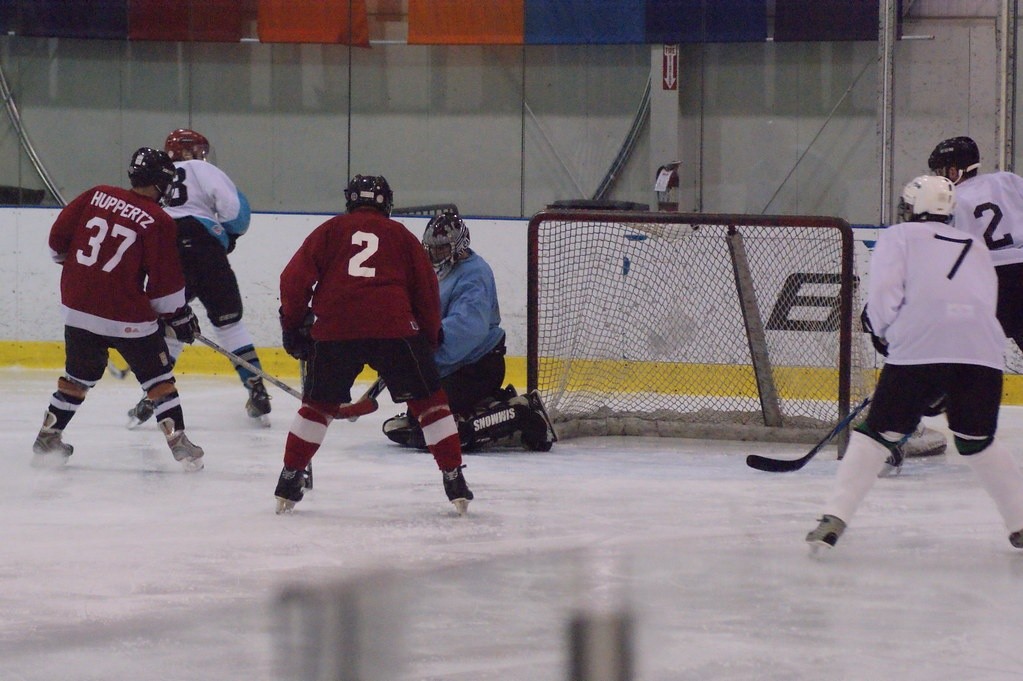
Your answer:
[221, 232, 240, 254]
[279, 303, 315, 361]
[860, 302, 889, 357]
[164, 305, 200, 344]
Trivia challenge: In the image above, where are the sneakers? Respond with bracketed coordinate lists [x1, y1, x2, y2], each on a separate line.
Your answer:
[245, 382, 273, 427]
[441, 463, 474, 516]
[1009, 529, 1023, 549]
[806, 514, 846, 560]
[273, 466, 306, 515]
[159, 418, 204, 472]
[33, 410, 74, 467]
[126, 395, 156, 430]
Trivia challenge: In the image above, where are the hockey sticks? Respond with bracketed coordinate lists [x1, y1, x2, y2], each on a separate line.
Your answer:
[105, 358, 132, 380]
[339, 379, 388, 422]
[746, 389, 875, 473]
[191, 332, 378, 420]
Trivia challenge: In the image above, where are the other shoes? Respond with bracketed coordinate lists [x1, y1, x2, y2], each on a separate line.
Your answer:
[517, 390, 555, 451]
[488, 384, 517, 448]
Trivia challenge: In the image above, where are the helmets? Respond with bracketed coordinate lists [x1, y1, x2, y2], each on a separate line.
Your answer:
[897, 176, 955, 223]
[422, 212, 470, 281]
[344, 174, 393, 218]
[164, 128, 209, 162]
[126, 147, 175, 186]
[928, 136, 980, 185]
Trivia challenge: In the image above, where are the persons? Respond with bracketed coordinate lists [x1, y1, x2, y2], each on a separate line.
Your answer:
[33, 148, 204, 472]
[382, 212, 558, 453]
[928, 137, 1023, 353]
[127, 127, 274, 429]
[274, 174, 474, 516]
[805, 174, 1023, 561]
